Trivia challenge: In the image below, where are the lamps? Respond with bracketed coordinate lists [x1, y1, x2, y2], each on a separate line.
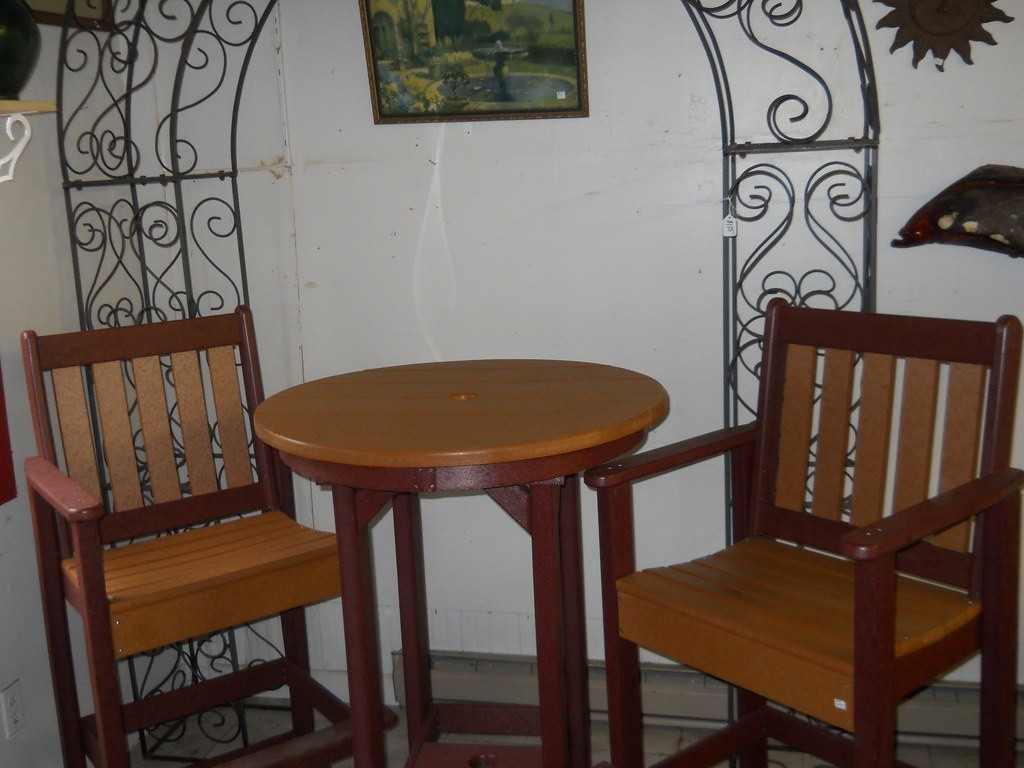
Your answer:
[890, 164, 1024, 259]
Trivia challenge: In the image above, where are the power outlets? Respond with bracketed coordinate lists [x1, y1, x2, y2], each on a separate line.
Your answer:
[0, 678, 25, 740]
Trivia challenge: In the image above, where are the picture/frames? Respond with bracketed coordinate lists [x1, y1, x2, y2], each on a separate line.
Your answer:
[358, 0, 588, 124]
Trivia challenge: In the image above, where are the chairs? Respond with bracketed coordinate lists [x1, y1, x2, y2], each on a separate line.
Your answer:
[581, 297, 1024, 768]
[22, 305, 398, 768]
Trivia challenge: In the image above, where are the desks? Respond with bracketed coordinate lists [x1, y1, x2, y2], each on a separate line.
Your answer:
[255, 360, 670, 768]
